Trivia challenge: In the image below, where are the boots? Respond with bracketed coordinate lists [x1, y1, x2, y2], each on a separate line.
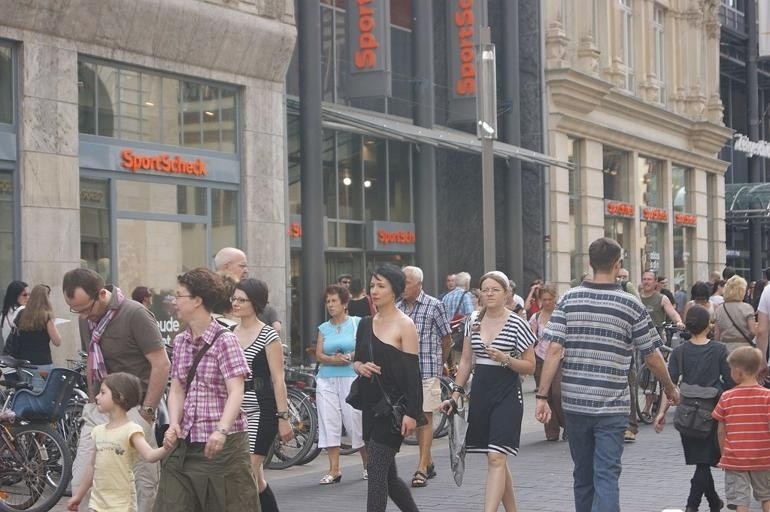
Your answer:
[260, 484, 280, 512]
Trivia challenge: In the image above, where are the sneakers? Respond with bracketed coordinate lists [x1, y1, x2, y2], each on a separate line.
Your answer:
[549, 436, 561, 441]
[625, 429, 636, 443]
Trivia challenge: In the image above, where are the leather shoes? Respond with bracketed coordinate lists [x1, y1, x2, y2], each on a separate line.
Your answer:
[319, 473, 342, 484]
[363, 469, 368, 480]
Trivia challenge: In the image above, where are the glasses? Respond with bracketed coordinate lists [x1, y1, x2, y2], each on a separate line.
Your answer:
[230, 297, 254, 303]
[68, 295, 98, 315]
[341, 281, 351, 284]
[18, 293, 29, 297]
[480, 287, 504, 294]
[175, 291, 196, 299]
[41, 284, 51, 293]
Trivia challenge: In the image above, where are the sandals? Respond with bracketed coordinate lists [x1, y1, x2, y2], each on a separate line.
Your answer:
[411, 472, 428, 487]
[428, 459, 436, 480]
[652, 394, 660, 417]
[638, 411, 650, 423]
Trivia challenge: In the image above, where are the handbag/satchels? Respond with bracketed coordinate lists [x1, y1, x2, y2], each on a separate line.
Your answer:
[368, 314, 429, 433]
[723, 303, 756, 351]
[155, 323, 228, 446]
[449, 403, 469, 486]
[448, 290, 470, 333]
[673, 346, 721, 440]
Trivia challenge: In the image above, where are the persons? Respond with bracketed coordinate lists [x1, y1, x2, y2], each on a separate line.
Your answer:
[257, 303, 282, 331]
[513, 293, 525, 308]
[10, 284, 62, 468]
[674, 283, 686, 314]
[315, 285, 368, 485]
[535, 237, 680, 512]
[524, 280, 544, 393]
[327, 273, 353, 321]
[638, 283, 643, 293]
[616, 277, 641, 300]
[151, 267, 261, 512]
[470, 288, 482, 310]
[713, 275, 755, 355]
[395, 266, 453, 487]
[437, 271, 457, 300]
[439, 270, 539, 512]
[581, 273, 593, 282]
[708, 280, 726, 306]
[711, 346, 770, 512]
[757, 284, 770, 385]
[654, 304, 737, 512]
[528, 284, 568, 442]
[0, 281, 31, 355]
[766, 267, 770, 284]
[504, 280, 523, 315]
[754, 279, 768, 311]
[441, 271, 474, 365]
[62, 268, 171, 512]
[705, 271, 721, 295]
[67, 372, 178, 512]
[535, 395, 548, 400]
[635, 269, 685, 422]
[346, 278, 376, 317]
[657, 276, 678, 346]
[616, 268, 629, 282]
[723, 267, 735, 281]
[214, 247, 249, 279]
[132, 286, 152, 306]
[210, 274, 238, 331]
[228, 277, 294, 512]
[748, 281, 756, 304]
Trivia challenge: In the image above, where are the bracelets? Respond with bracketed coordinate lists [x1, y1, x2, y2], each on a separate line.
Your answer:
[502, 355, 510, 368]
[347, 354, 352, 362]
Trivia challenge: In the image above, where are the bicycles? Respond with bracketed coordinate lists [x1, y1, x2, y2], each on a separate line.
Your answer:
[265, 362, 359, 470]
[636, 321, 691, 425]
[0, 352, 102, 511]
[404, 374, 457, 443]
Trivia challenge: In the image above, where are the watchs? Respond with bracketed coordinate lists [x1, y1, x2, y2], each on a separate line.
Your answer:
[536, 394, 548, 399]
[454, 386, 464, 393]
[217, 427, 231, 435]
[142, 405, 155, 415]
[276, 411, 290, 420]
